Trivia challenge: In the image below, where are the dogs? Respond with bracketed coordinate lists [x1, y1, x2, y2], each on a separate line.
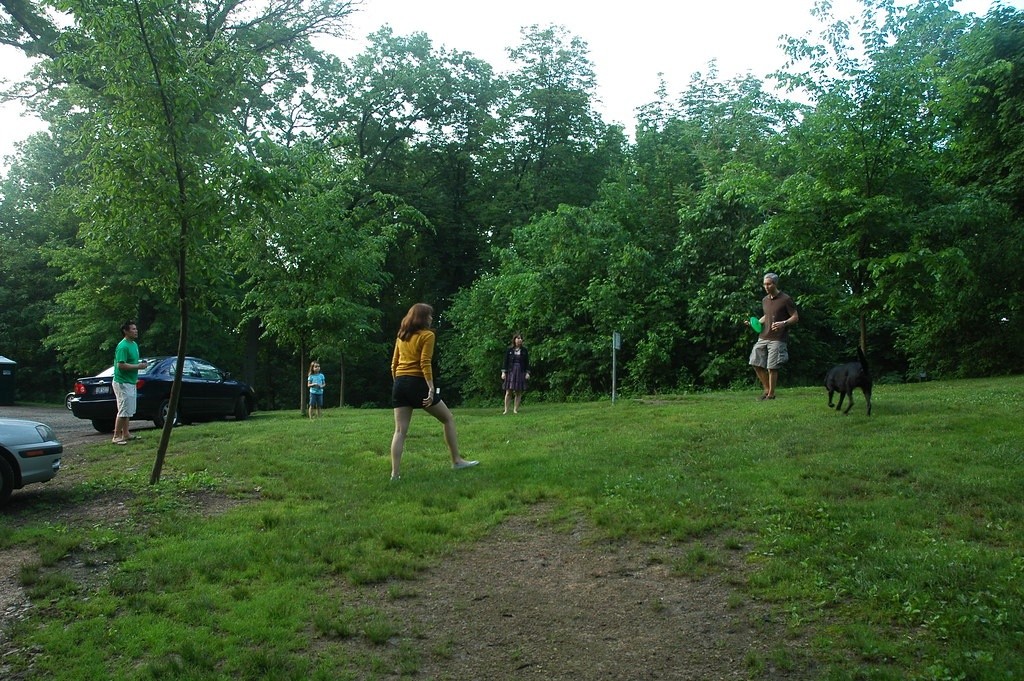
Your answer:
[822, 344, 872, 418]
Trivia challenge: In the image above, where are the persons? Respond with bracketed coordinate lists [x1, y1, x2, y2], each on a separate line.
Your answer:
[307, 361, 326, 421]
[388, 302, 479, 480]
[501, 333, 531, 414]
[744, 272, 799, 403]
[111, 319, 148, 446]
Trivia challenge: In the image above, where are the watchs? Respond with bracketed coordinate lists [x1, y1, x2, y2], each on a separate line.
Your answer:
[784, 320, 788, 327]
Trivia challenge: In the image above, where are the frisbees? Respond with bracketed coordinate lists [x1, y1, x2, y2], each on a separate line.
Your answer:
[750, 317, 762, 333]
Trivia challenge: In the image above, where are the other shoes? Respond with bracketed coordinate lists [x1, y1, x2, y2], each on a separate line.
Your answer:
[452, 459, 479, 469]
[756, 393, 776, 401]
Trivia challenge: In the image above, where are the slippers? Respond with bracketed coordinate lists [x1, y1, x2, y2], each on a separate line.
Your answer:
[122, 435, 141, 440]
[111, 439, 127, 444]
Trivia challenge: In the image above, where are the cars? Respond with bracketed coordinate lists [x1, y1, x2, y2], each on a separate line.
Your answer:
[71, 353, 257, 430]
[0, 414, 65, 514]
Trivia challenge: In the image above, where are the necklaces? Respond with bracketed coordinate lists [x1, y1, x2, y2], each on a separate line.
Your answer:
[514, 347, 520, 352]
